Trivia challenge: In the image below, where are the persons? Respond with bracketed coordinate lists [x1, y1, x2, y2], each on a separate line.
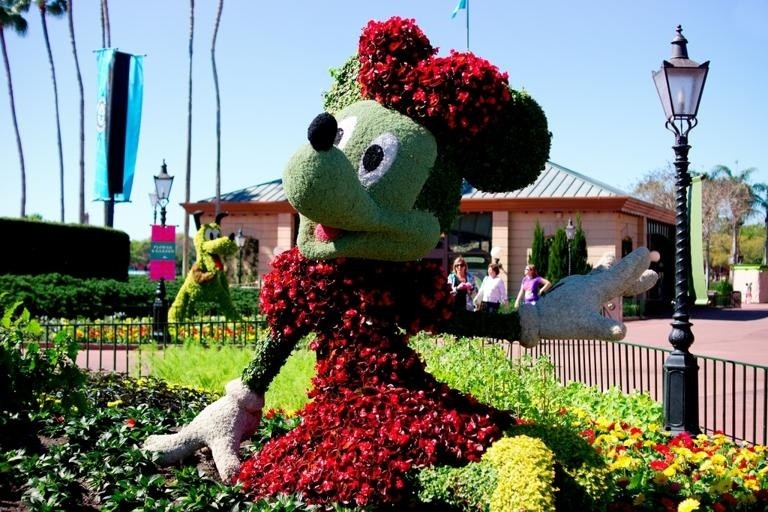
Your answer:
[473, 262, 509, 313]
[514, 264, 552, 310]
[447, 256, 478, 312]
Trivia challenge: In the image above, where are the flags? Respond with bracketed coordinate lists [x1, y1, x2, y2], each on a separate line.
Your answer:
[448, 0, 466, 17]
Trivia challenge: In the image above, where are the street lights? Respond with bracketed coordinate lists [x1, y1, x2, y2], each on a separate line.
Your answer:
[566, 217, 576, 276]
[148, 159, 175, 342]
[651, 24, 711, 436]
